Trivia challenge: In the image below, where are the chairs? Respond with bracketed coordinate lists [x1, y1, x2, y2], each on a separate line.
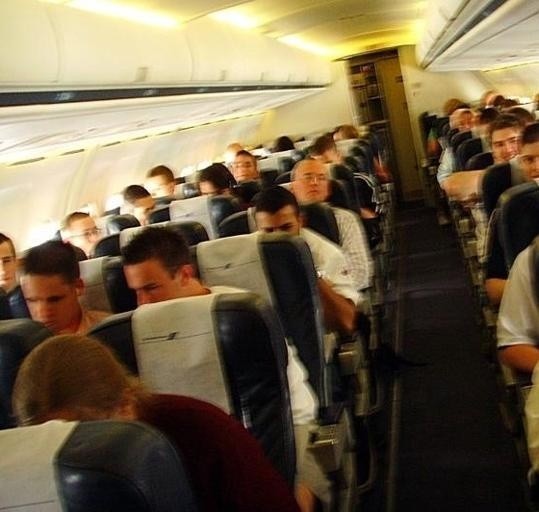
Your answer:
[0, 123, 393, 512]
[416, 95, 538, 510]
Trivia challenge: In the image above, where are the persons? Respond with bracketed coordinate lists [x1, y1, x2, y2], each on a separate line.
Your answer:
[1, 125, 389, 512]
[425, 93, 538, 510]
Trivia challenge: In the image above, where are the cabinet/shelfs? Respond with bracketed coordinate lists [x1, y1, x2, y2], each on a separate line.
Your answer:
[348, 61, 386, 123]
